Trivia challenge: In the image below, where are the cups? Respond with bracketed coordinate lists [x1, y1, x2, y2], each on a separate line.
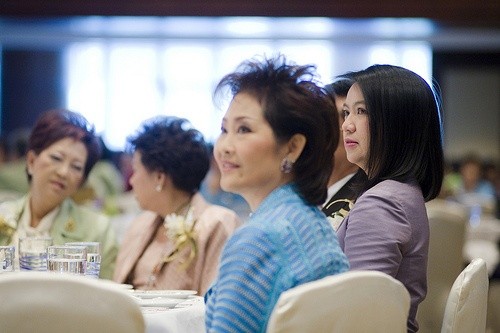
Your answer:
[0, 246, 15, 274]
[47, 246, 87, 276]
[65, 242, 101, 279]
[18, 237, 54, 272]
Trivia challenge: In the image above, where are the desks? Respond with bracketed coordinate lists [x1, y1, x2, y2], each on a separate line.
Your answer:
[128, 289, 207, 333]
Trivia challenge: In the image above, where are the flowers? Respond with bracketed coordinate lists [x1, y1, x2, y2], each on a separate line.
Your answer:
[163, 213, 201, 279]
[327, 199, 354, 232]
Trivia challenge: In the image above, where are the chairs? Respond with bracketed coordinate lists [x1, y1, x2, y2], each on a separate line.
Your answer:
[416, 200, 467, 333]
[0, 271, 147, 333]
[439, 258, 489, 333]
[264, 269, 412, 333]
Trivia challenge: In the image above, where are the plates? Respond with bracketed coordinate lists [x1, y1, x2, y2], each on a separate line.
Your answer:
[134, 291, 199, 297]
[137, 299, 180, 306]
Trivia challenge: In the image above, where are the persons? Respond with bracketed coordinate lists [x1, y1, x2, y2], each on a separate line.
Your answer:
[335, 62, 445, 333]
[113, 115, 244, 295]
[0, 107, 118, 282]
[316, 80, 370, 229]
[202, 49, 350, 333]
